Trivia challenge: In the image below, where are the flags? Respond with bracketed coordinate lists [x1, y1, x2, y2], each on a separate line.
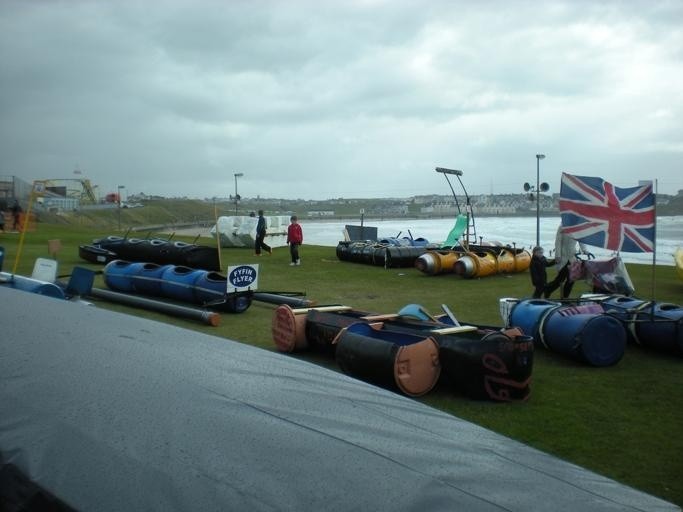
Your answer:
[560, 172, 655, 254]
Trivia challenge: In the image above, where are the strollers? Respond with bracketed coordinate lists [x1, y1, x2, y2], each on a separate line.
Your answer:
[574, 252, 635, 296]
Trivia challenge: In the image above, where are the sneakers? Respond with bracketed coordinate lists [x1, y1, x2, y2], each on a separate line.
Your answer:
[290, 263, 295, 266]
[296, 258, 300, 265]
[270, 247, 272, 255]
[254, 253, 262, 256]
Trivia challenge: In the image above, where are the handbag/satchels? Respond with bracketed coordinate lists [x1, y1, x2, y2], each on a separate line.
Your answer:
[570, 262, 591, 280]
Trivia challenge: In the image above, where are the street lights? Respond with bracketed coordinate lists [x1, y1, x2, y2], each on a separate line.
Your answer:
[524, 155, 549, 247]
[359, 208, 365, 226]
[118, 186, 125, 230]
[230, 173, 243, 216]
[464, 198, 472, 252]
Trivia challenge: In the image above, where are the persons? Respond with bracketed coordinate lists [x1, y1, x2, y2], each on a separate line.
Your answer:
[543, 224, 595, 306]
[0, 213, 5, 231]
[249, 212, 255, 217]
[7, 200, 25, 231]
[252, 210, 271, 256]
[285, 215, 303, 266]
[528, 246, 560, 299]
